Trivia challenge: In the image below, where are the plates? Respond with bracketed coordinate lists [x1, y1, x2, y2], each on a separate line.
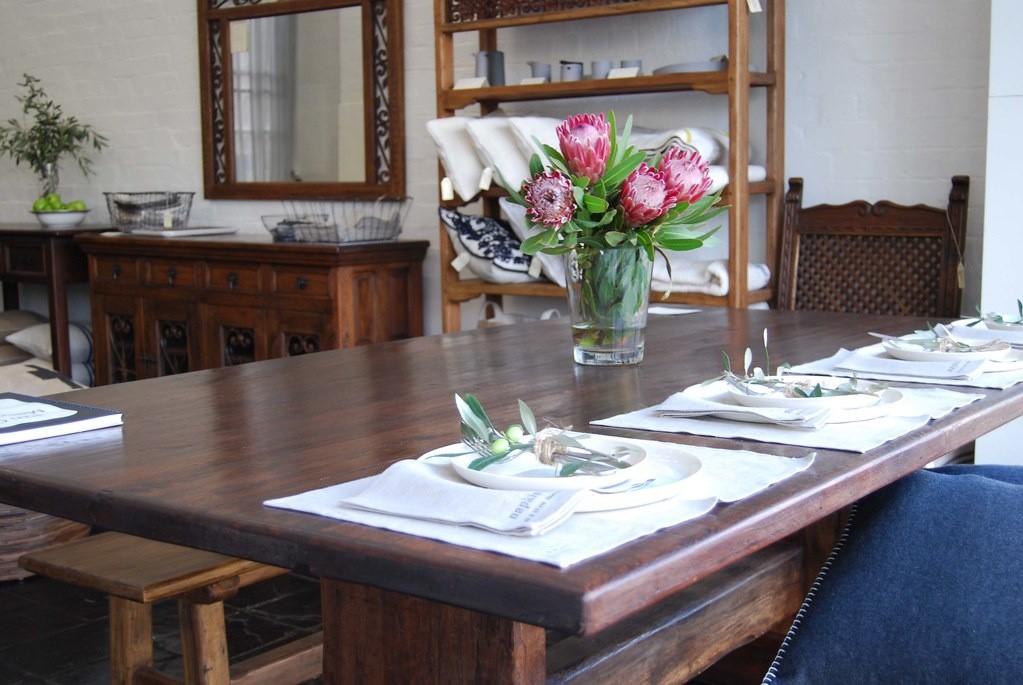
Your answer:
[725, 375, 887, 409]
[951, 318, 988, 329]
[418, 442, 703, 514]
[984, 322, 1023, 331]
[682, 375, 904, 424]
[652, 61, 727, 76]
[881, 338, 1012, 360]
[851, 343, 1023, 372]
[451, 437, 649, 491]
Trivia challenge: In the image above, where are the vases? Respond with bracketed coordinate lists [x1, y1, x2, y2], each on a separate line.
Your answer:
[38, 163, 59, 199]
[561, 247, 652, 364]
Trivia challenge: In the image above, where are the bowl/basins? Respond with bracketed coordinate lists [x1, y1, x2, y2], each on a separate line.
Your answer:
[29, 209, 92, 226]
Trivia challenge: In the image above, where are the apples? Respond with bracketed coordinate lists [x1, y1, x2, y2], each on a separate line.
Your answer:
[33, 193, 85, 211]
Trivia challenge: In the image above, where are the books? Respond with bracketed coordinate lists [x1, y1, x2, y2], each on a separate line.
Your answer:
[132, 226, 237, 238]
[0, 392, 125, 445]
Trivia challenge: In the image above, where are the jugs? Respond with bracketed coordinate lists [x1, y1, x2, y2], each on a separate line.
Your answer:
[472, 51, 506, 86]
[525, 60, 551, 83]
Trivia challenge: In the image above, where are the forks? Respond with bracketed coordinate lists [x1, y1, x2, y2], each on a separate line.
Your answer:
[722, 376, 789, 396]
[460, 433, 632, 469]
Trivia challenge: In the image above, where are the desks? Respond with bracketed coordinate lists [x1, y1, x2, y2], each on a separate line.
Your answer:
[0, 305, 1023, 685]
[0, 222, 120, 378]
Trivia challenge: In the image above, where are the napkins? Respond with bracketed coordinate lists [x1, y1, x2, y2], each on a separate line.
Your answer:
[952, 325, 1023, 347]
[834, 350, 1017, 382]
[653, 377, 888, 428]
[349, 451, 654, 536]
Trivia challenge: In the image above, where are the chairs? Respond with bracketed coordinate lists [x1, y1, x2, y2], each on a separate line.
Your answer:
[777, 174, 973, 497]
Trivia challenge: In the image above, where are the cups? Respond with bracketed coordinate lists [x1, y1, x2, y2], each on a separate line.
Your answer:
[620, 60, 643, 77]
[591, 60, 613, 79]
[560, 61, 583, 81]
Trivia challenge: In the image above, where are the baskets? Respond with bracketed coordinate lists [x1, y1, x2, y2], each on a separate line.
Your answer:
[260, 192, 413, 243]
[103, 190, 196, 233]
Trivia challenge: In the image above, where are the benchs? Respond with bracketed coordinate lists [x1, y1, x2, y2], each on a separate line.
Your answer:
[16, 529, 324, 685]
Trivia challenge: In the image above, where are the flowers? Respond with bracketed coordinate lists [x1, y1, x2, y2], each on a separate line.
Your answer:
[492, 112, 734, 338]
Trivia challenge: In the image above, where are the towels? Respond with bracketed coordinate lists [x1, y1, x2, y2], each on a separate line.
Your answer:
[617, 125, 767, 196]
[649, 258, 771, 297]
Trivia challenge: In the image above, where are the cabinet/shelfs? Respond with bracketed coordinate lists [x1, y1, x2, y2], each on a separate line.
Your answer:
[434, 0, 786, 334]
[77, 229, 431, 386]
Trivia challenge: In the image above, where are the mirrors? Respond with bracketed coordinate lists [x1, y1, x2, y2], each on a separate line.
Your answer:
[196, 0, 406, 201]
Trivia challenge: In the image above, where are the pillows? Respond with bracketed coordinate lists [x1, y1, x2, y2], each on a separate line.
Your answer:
[1, 309, 93, 398]
[428, 115, 751, 284]
[762, 463, 1023, 685]
